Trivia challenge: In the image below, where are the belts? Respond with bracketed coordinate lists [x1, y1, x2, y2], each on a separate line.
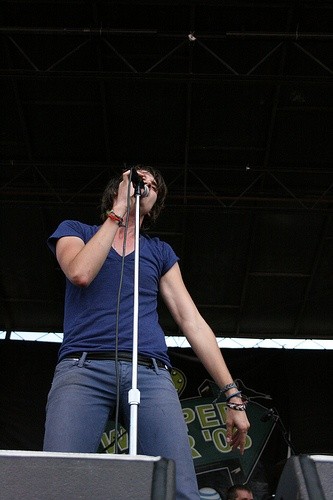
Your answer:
[65, 351, 165, 368]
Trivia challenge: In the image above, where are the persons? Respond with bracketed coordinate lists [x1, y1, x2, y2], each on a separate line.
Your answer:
[226, 484, 253, 500]
[42, 162, 250, 500]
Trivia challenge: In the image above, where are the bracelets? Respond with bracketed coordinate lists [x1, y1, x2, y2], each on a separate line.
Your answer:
[220, 383, 246, 411]
[105, 209, 123, 223]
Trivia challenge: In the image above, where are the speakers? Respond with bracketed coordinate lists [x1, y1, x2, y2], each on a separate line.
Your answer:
[0, 448, 176, 500]
[273, 454, 333, 500]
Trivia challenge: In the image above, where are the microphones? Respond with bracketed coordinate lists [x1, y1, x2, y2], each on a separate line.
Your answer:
[130, 167, 150, 197]
